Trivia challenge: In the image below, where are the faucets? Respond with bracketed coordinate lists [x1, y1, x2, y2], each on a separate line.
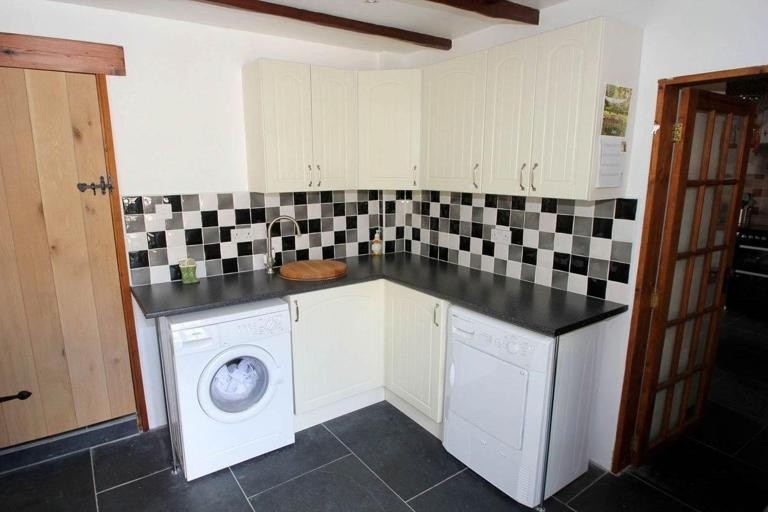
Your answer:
[263, 215, 301, 274]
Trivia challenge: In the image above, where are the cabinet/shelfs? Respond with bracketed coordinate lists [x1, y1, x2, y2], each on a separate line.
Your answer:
[488, 16, 645, 202]
[239, 56, 358, 194]
[359, 48, 486, 194]
[283, 279, 451, 443]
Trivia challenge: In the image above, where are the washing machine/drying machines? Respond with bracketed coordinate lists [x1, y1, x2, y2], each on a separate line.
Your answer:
[154, 299, 297, 483]
[442, 303, 603, 506]
